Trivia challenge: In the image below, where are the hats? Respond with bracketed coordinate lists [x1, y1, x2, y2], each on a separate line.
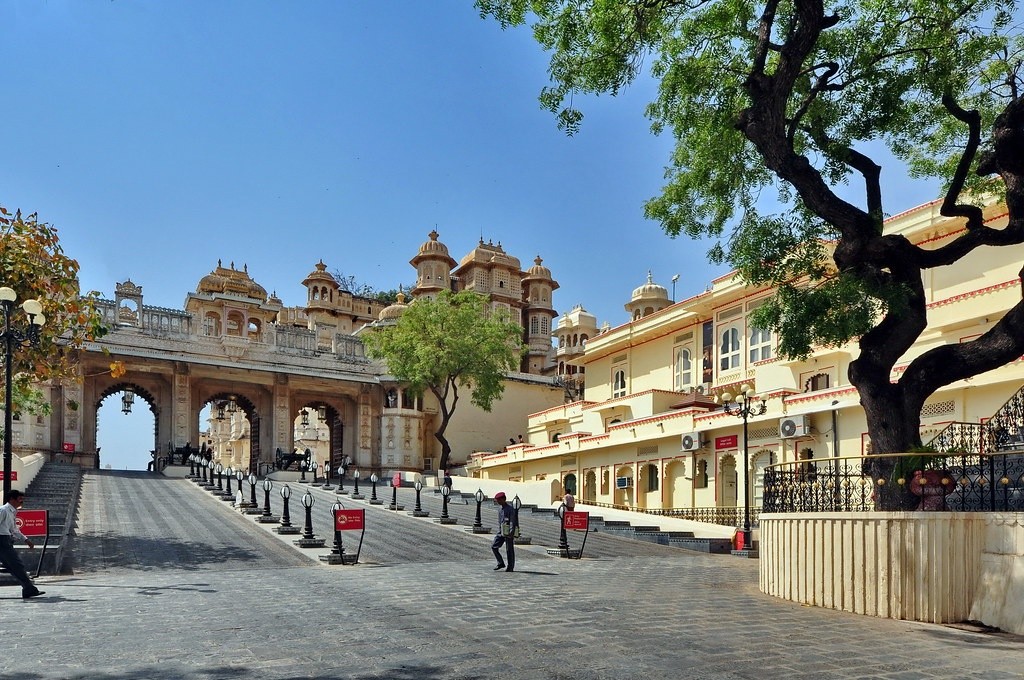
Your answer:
[444, 473, 449, 476]
[495, 492, 506, 499]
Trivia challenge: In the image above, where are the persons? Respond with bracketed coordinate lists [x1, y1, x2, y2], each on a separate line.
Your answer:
[0, 490, 46, 599]
[491, 492, 516, 572]
[564, 489, 575, 512]
[518, 435, 524, 443]
[510, 439, 516, 445]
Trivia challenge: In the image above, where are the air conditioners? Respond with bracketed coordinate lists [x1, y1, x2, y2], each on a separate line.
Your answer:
[616, 477, 632, 488]
[682, 431, 701, 451]
[694, 382, 711, 395]
[675, 386, 694, 395]
[780, 414, 811, 438]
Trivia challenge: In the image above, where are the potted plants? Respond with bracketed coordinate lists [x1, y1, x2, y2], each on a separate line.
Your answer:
[887, 442, 970, 512]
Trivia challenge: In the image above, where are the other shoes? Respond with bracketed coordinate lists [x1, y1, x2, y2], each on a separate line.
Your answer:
[493, 563, 506, 570]
[505, 565, 513, 572]
[21, 586, 46, 599]
